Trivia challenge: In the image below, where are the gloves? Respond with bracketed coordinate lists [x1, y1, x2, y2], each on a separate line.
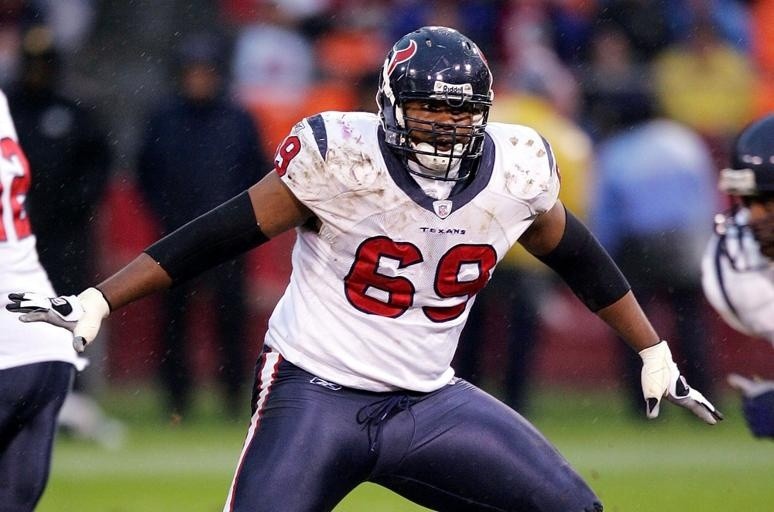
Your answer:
[638, 339, 725, 426]
[6, 285, 112, 354]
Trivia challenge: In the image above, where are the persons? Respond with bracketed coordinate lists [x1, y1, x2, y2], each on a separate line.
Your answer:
[0, 0, 774, 441]
[0, 88, 84, 512]
[3, 24, 723, 511]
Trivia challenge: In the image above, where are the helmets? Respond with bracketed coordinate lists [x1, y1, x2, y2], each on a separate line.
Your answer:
[714, 114, 774, 273]
[376, 25, 494, 183]
